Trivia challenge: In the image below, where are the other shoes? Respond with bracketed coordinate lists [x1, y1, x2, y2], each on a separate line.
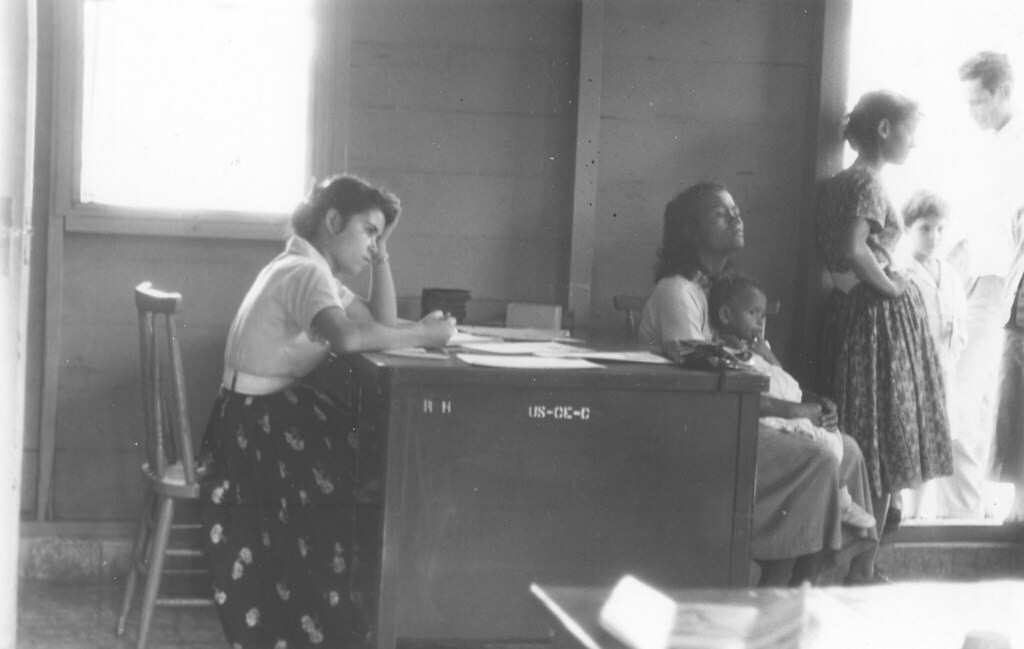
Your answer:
[842, 564, 895, 587]
[841, 502, 877, 527]
[887, 506, 902, 523]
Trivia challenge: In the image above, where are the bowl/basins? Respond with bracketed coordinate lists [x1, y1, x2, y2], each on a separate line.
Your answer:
[421, 288, 470, 322]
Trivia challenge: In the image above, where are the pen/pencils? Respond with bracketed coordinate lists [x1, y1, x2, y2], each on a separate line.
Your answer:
[442, 311, 451, 319]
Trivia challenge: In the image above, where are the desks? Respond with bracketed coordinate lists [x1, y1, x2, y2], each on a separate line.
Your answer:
[336, 325, 772, 649]
[525, 581, 1024, 649]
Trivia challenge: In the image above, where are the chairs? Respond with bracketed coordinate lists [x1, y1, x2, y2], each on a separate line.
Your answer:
[613, 293, 781, 340]
[113, 281, 201, 648]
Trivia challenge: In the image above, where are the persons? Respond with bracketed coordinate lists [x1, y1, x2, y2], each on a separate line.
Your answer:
[817, 52, 1024, 585]
[196, 176, 456, 649]
[637, 181, 879, 587]
